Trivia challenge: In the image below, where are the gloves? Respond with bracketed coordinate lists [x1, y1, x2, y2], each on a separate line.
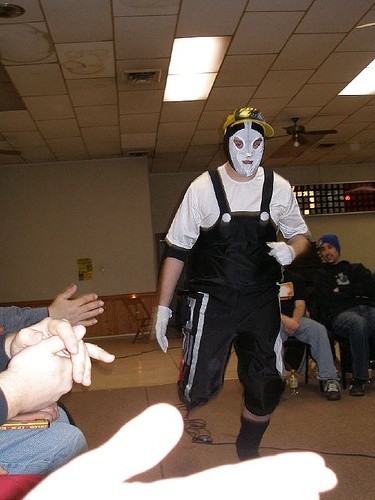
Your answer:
[154, 304, 172, 354]
[265, 241, 296, 267]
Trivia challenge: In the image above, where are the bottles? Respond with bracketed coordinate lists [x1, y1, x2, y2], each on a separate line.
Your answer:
[289, 369, 299, 396]
[368, 361, 374, 380]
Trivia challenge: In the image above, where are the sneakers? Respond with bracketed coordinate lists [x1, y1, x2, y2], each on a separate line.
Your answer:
[322, 379, 341, 401]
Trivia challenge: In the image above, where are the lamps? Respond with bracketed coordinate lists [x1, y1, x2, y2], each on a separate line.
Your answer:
[292, 134, 302, 146]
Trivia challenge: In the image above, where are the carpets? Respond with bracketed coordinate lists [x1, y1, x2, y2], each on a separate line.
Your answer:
[59, 372, 375, 500]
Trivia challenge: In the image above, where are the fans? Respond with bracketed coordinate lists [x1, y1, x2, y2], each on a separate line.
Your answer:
[262, 118, 338, 140]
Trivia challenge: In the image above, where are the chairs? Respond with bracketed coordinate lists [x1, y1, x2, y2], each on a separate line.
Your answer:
[119, 296, 155, 343]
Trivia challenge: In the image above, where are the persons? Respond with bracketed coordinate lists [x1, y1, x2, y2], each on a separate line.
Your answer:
[22, 403, 338, 500]
[0, 317, 116, 428]
[309, 234, 375, 396]
[280, 266, 343, 400]
[0, 285, 104, 475]
[156, 106, 312, 463]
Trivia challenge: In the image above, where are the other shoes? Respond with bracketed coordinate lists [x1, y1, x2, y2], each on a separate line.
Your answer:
[348, 379, 366, 396]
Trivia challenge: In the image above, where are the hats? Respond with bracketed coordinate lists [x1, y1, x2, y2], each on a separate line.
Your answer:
[222, 107, 274, 138]
[318, 234, 340, 256]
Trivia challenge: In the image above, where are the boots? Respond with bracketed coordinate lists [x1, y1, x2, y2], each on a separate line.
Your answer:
[236, 413, 271, 461]
